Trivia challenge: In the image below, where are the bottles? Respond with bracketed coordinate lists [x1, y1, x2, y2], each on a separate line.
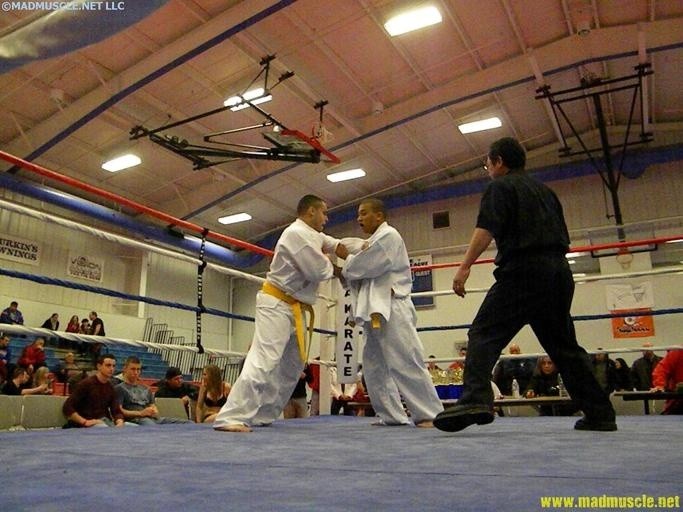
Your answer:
[511, 376, 519, 398]
[559, 374, 568, 397]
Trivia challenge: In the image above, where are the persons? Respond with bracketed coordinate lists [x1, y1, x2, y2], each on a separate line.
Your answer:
[0, 299, 120, 398]
[195, 364, 230, 424]
[330, 197, 443, 427]
[427, 343, 682, 417]
[432, 136, 618, 432]
[309, 355, 375, 419]
[280, 361, 313, 418]
[110, 355, 194, 426]
[213, 194, 368, 433]
[61, 354, 140, 428]
[150, 366, 200, 406]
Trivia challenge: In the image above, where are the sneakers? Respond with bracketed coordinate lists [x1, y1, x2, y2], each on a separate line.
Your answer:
[432, 399, 496, 432]
[574, 415, 617, 431]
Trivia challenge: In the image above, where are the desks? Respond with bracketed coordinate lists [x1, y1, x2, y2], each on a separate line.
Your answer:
[435, 383, 465, 398]
[347, 393, 577, 419]
[613, 386, 682, 414]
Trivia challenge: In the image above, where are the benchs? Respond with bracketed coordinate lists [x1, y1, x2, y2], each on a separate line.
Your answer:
[0, 321, 201, 398]
